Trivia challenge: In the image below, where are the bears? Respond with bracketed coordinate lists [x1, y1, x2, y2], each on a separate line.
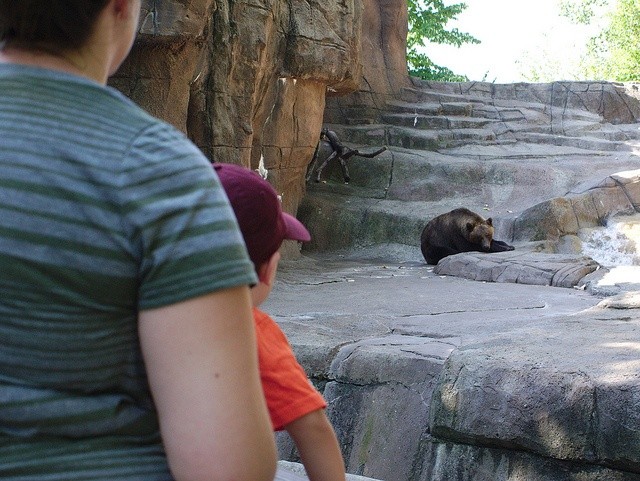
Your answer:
[420, 208, 515, 265]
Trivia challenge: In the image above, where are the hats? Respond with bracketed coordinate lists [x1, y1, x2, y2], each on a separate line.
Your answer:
[214, 162, 311, 263]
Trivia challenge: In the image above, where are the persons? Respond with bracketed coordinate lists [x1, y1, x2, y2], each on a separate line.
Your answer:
[1, 0, 277, 480]
[210, 162, 347, 480]
